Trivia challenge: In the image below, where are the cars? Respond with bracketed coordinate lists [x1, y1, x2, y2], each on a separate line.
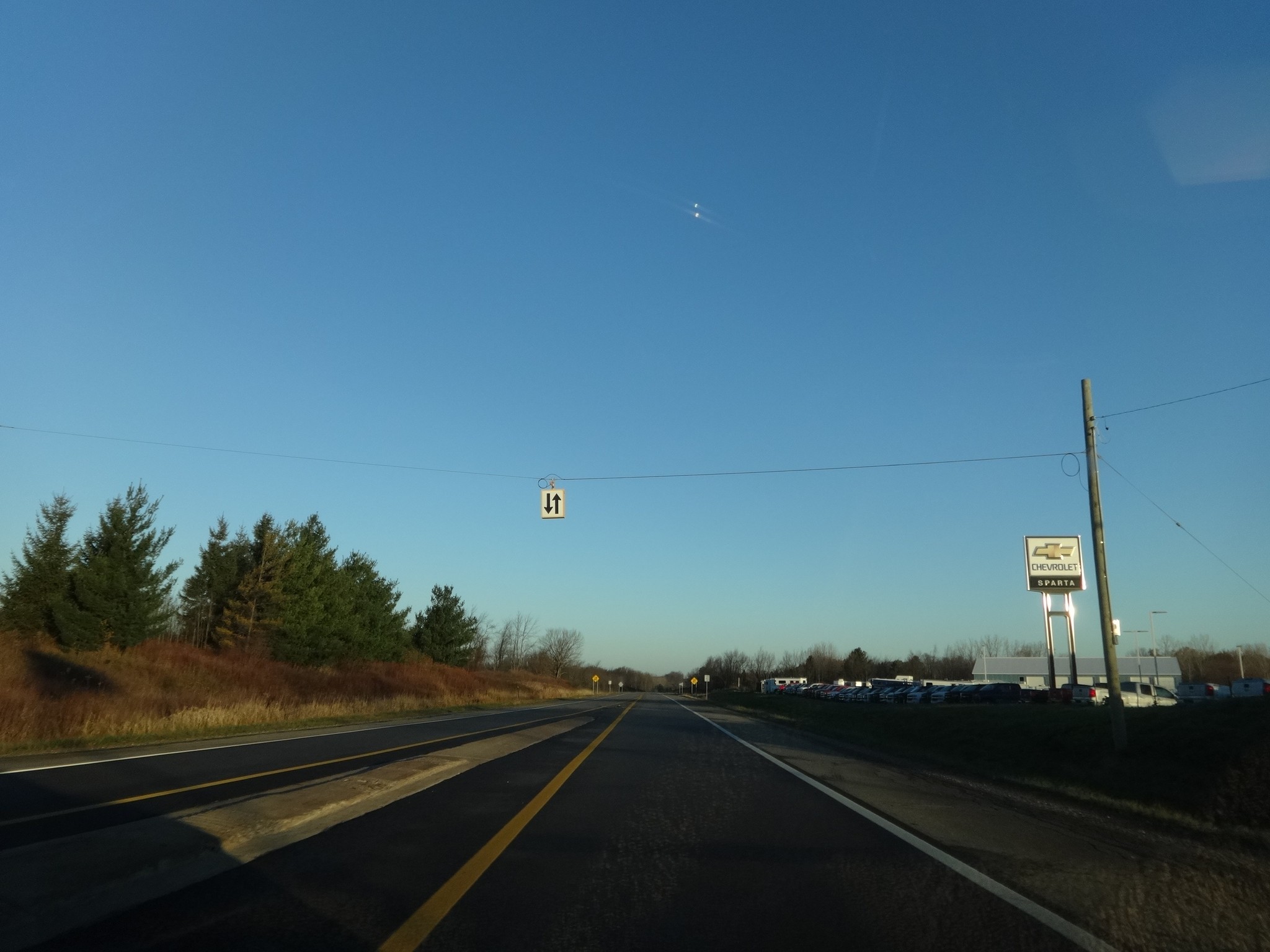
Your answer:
[762, 677, 1270, 708]
